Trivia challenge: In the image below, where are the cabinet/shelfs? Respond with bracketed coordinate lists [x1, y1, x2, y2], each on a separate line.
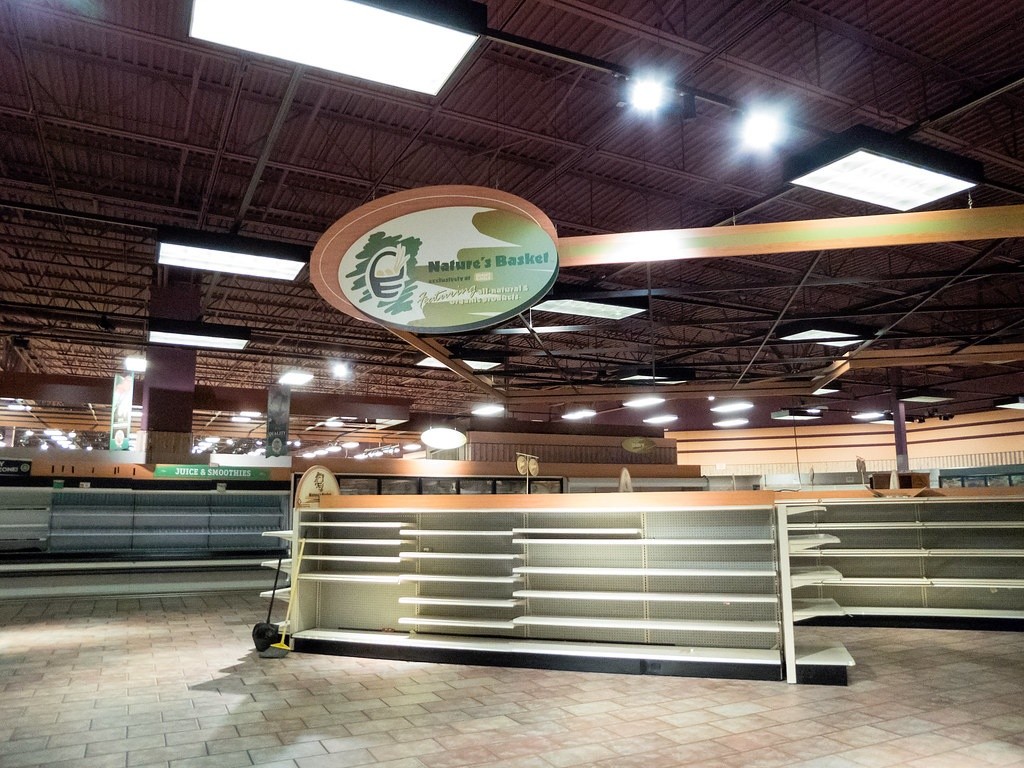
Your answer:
[0, 456, 292, 601]
[250, 490, 856, 686]
[787, 486, 1024, 632]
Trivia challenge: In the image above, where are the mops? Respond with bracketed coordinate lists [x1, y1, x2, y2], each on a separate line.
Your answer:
[258, 538, 306, 658]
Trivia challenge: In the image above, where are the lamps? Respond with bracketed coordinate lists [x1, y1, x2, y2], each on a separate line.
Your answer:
[274, 365, 316, 389]
[770, 408, 824, 421]
[813, 380, 842, 396]
[611, 70, 630, 108]
[895, 388, 958, 403]
[530, 282, 649, 320]
[148, 317, 254, 352]
[618, 368, 693, 384]
[413, 342, 505, 371]
[993, 395, 1024, 410]
[677, 82, 699, 120]
[153, 225, 309, 284]
[781, 122, 984, 212]
[775, 312, 877, 348]
[870, 414, 915, 426]
[187, 0, 486, 99]
